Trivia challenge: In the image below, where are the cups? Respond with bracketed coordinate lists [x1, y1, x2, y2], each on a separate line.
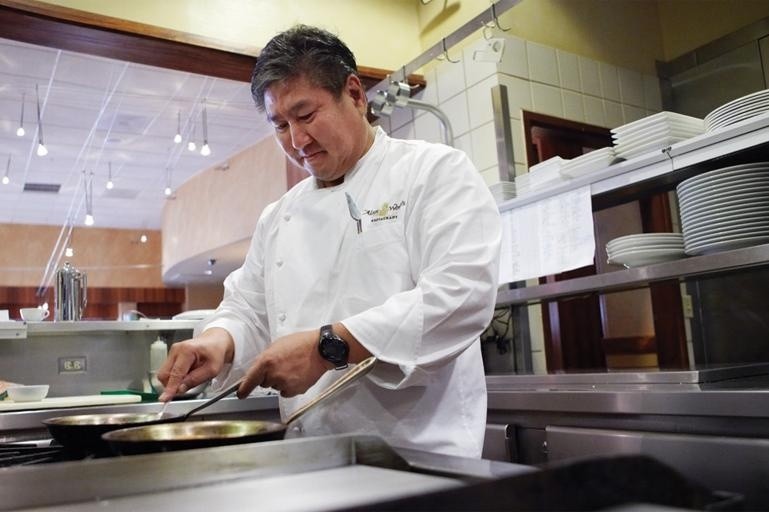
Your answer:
[19, 306, 50, 322]
[118, 300, 140, 322]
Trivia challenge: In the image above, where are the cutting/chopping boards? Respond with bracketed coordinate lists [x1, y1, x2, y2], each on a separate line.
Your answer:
[2, 395, 142, 413]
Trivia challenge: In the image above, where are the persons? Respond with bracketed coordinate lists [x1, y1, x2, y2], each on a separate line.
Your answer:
[156, 23, 501, 459]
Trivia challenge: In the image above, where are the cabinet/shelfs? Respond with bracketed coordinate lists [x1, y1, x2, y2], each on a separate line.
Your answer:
[470, 106, 768, 425]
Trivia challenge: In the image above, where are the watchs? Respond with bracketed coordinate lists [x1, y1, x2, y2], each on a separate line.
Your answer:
[319, 324, 351, 372]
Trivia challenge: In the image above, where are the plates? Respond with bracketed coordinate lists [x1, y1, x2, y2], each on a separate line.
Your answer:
[605, 231, 682, 272]
[514, 155, 571, 199]
[703, 89, 769, 134]
[558, 146, 620, 178]
[676, 161, 769, 260]
[487, 181, 516, 202]
[610, 110, 703, 164]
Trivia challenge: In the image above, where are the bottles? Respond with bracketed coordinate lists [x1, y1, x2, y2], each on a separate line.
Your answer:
[53, 262, 86, 322]
[149, 336, 169, 371]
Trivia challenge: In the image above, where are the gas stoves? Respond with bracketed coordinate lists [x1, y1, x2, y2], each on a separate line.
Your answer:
[0, 433, 138, 470]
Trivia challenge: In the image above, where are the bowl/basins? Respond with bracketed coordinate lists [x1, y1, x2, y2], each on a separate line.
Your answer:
[7, 384, 49, 402]
[148, 368, 211, 399]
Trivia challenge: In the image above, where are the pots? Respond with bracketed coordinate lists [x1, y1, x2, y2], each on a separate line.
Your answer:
[108, 359, 381, 450]
[42, 373, 243, 448]
